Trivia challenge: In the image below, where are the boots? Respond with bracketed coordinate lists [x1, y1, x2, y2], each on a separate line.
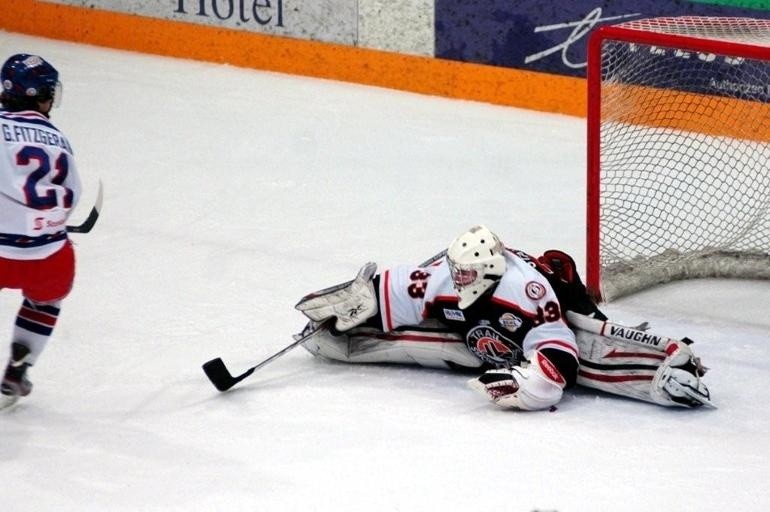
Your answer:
[0, 342, 35, 396]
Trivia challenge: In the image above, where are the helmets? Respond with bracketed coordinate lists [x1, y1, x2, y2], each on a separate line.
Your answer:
[446, 226, 508, 311]
[0, 53, 60, 99]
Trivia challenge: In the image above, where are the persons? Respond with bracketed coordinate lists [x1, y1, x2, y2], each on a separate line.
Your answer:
[2, 54, 83, 396]
[290, 221, 711, 412]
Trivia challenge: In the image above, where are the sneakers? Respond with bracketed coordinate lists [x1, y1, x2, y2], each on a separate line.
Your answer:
[301, 321, 320, 354]
[663, 365, 711, 406]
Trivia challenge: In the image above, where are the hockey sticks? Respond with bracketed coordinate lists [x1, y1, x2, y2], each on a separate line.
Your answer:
[202, 249, 449, 391]
[66, 179, 104, 233]
